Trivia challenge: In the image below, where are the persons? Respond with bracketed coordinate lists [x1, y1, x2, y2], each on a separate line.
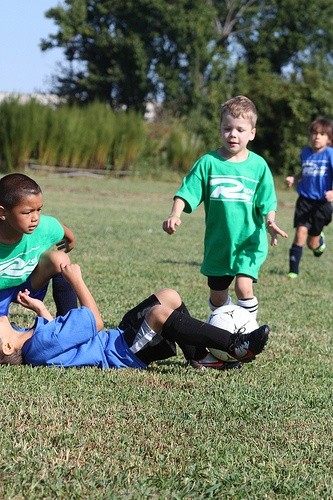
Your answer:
[0, 262, 269, 370]
[0, 173, 77, 318]
[285, 118, 333, 279]
[163, 96, 288, 320]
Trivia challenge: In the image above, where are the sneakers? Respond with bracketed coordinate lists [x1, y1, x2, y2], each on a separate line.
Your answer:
[313, 232, 325, 257]
[227, 325, 269, 361]
[288, 272, 298, 278]
[200, 353, 242, 369]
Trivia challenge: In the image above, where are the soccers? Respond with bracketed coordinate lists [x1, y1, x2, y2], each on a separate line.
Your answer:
[207, 304, 260, 362]
